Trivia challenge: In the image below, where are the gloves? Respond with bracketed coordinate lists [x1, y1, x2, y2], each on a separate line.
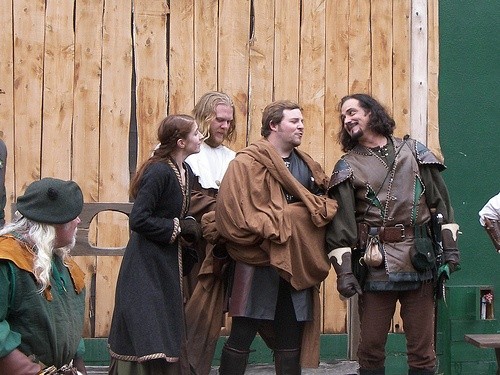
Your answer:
[441, 223, 461, 272]
[179, 219, 203, 242]
[328, 247, 362, 298]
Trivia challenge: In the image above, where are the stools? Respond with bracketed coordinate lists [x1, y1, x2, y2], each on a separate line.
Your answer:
[464, 333, 500, 375]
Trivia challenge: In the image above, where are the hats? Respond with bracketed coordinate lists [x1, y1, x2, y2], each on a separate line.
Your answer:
[17, 177, 83, 224]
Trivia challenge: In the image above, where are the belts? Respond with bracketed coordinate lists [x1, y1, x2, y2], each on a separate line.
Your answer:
[368, 226, 416, 242]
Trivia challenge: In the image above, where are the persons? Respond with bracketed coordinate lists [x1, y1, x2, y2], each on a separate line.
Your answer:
[107, 114, 205, 375]
[215, 99, 338, 375]
[0, 177, 87, 375]
[151, 90, 237, 375]
[326, 93, 462, 375]
[0, 138, 8, 230]
[478, 191, 500, 253]
